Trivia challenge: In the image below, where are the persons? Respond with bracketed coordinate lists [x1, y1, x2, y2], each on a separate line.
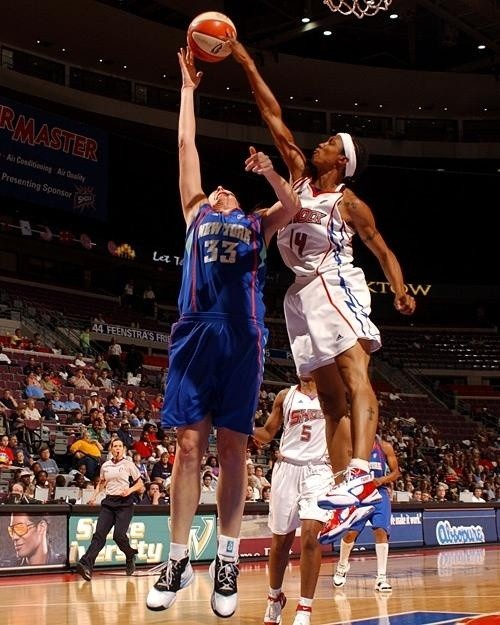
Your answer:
[75, 581, 139, 625]
[146, 45, 301, 618]
[1, 312, 500, 506]
[334, 432, 400, 591]
[5, 515, 66, 567]
[249, 374, 337, 624]
[334, 589, 392, 625]
[125, 280, 134, 308]
[143, 285, 155, 317]
[221, 29, 418, 545]
[73, 438, 138, 582]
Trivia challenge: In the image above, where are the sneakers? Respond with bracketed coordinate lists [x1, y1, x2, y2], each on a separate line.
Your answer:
[292, 604, 312, 625]
[317, 464, 383, 509]
[264, 592, 287, 625]
[332, 560, 350, 588]
[146, 549, 194, 611]
[208, 554, 240, 618]
[126, 554, 136, 575]
[316, 505, 376, 545]
[375, 575, 393, 592]
[76, 562, 91, 581]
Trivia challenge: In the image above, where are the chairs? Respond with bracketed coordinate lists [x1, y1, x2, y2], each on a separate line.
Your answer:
[0, 280, 500, 504]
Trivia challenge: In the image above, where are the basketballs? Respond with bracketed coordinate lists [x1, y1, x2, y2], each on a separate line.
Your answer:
[188, 12, 237, 62]
